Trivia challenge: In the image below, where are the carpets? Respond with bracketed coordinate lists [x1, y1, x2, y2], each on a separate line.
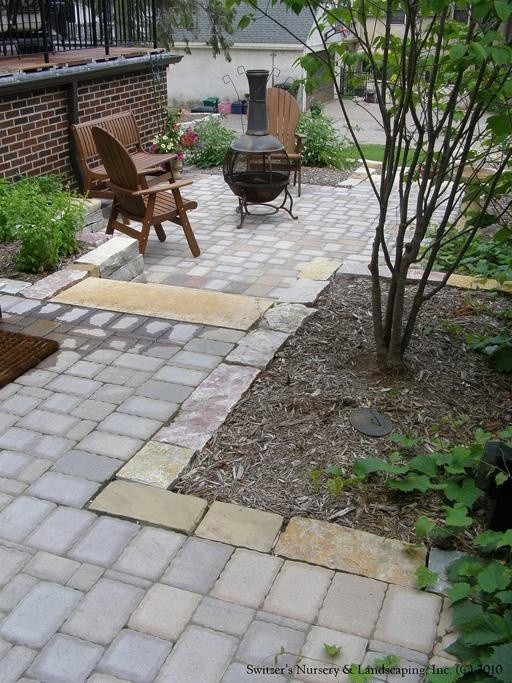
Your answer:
[0, 328, 59, 390]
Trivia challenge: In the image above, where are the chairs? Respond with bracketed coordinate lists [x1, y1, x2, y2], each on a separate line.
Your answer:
[89, 125, 200, 258]
[246, 86, 309, 198]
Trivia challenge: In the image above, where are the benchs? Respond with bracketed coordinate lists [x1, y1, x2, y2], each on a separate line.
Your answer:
[71, 110, 179, 224]
